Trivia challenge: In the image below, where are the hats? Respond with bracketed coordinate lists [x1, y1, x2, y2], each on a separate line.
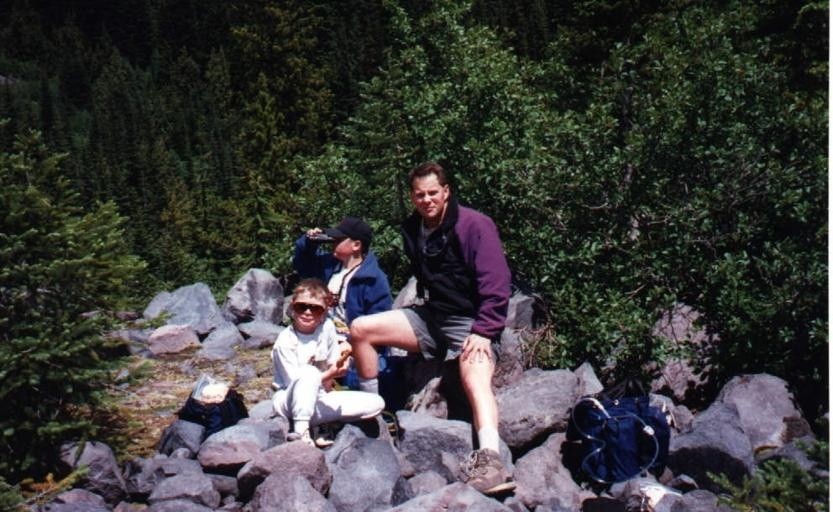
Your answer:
[326, 217, 372, 246]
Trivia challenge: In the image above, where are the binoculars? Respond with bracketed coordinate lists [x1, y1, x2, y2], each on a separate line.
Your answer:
[306, 233, 336, 241]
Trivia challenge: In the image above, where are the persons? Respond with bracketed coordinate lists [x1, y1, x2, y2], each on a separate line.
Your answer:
[290, 217, 395, 391]
[347, 162, 517, 495]
[270, 275, 386, 447]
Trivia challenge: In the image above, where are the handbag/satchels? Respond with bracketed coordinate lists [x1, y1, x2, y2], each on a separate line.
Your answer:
[566, 396, 671, 489]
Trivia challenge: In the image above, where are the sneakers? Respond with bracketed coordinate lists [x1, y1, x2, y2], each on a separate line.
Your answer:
[468, 449, 507, 491]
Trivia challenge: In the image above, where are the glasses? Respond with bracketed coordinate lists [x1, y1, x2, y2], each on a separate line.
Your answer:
[291, 301, 326, 316]
